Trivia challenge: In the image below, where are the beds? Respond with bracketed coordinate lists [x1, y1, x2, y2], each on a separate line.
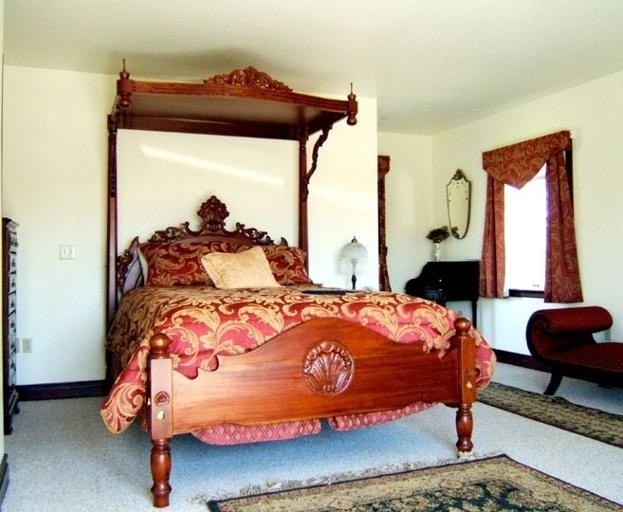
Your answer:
[99, 59, 499, 508]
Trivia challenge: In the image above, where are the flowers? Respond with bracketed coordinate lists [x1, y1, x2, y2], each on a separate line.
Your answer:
[425, 225, 451, 243]
[452, 226, 460, 238]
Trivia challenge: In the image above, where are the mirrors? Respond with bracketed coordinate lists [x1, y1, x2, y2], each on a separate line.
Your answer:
[445, 169, 471, 239]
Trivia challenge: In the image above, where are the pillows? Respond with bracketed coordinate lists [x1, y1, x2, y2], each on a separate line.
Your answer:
[136, 239, 314, 292]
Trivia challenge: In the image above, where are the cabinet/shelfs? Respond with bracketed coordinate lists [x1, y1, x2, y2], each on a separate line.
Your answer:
[3, 217, 21, 436]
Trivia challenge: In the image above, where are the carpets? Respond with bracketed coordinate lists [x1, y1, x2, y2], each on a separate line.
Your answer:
[475, 381, 623, 448]
[207, 453, 622, 511]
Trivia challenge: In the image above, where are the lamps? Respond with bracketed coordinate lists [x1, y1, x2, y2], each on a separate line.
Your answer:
[340, 235, 369, 290]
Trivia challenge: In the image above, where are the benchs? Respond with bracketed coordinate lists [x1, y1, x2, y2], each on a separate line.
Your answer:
[525, 305, 623, 396]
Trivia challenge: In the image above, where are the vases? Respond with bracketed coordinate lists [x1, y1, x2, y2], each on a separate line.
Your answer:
[432, 242, 441, 262]
